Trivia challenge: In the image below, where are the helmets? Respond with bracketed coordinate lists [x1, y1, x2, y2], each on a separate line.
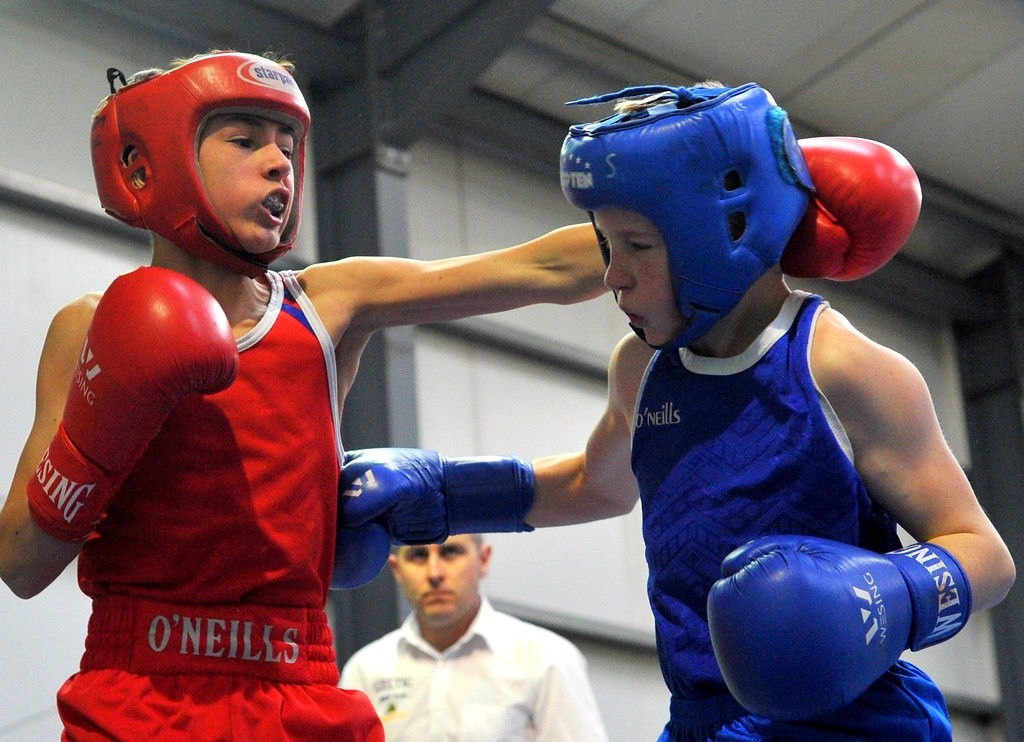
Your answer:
[89, 50, 312, 278]
[559, 82, 816, 354]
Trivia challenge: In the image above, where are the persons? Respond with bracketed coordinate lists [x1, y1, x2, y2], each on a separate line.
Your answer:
[339, 79, 1016, 742]
[0, 48, 923, 742]
[338, 530, 611, 740]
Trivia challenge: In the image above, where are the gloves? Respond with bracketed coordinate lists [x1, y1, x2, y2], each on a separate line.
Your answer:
[26, 265, 239, 545]
[780, 136, 922, 283]
[707, 532, 972, 722]
[329, 446, 536, 591]
